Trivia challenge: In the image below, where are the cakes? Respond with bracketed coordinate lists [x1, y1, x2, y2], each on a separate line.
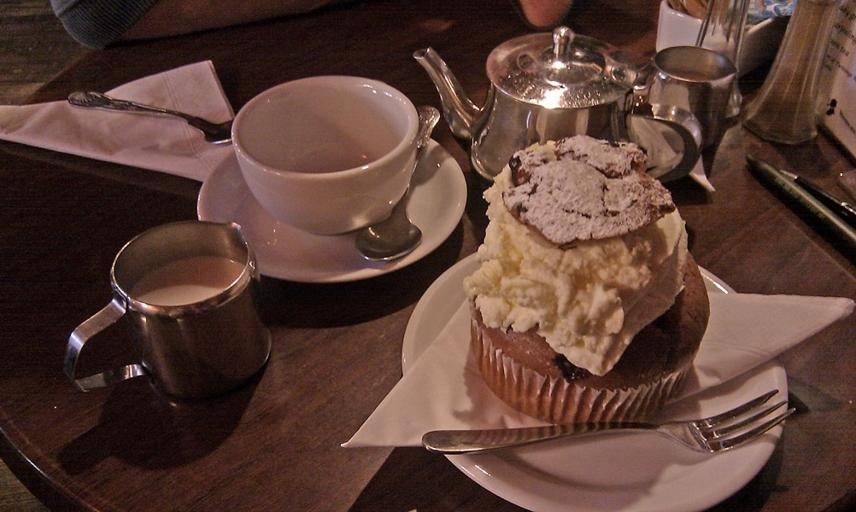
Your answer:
[465, 134, 711, 428]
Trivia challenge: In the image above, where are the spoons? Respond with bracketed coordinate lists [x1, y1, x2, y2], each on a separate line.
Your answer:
[355, 105, 439, 264]
[71, 89, 232, 143]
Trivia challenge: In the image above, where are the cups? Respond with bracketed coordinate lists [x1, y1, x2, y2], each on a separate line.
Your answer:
[65, 220, 273, 408]
[655, 44, 739, 153]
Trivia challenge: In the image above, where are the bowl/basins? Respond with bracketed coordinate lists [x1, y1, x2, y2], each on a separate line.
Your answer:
[232, 76, 420, 238]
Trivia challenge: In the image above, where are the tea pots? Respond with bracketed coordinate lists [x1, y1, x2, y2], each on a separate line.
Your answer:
[414, 27, 705, 191]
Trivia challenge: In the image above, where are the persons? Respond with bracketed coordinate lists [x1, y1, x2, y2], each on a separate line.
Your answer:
[48, 0, 575, 53]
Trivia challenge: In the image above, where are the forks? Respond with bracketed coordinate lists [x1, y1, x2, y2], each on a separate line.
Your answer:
[421, 389, 797, 460]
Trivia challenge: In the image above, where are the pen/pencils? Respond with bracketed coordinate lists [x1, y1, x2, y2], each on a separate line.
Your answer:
[745, 154, 856, 262]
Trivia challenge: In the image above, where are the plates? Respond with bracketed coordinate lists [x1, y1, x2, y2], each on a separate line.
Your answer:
[400, 252, 791, 512]
[198, 127, 468, 283]
